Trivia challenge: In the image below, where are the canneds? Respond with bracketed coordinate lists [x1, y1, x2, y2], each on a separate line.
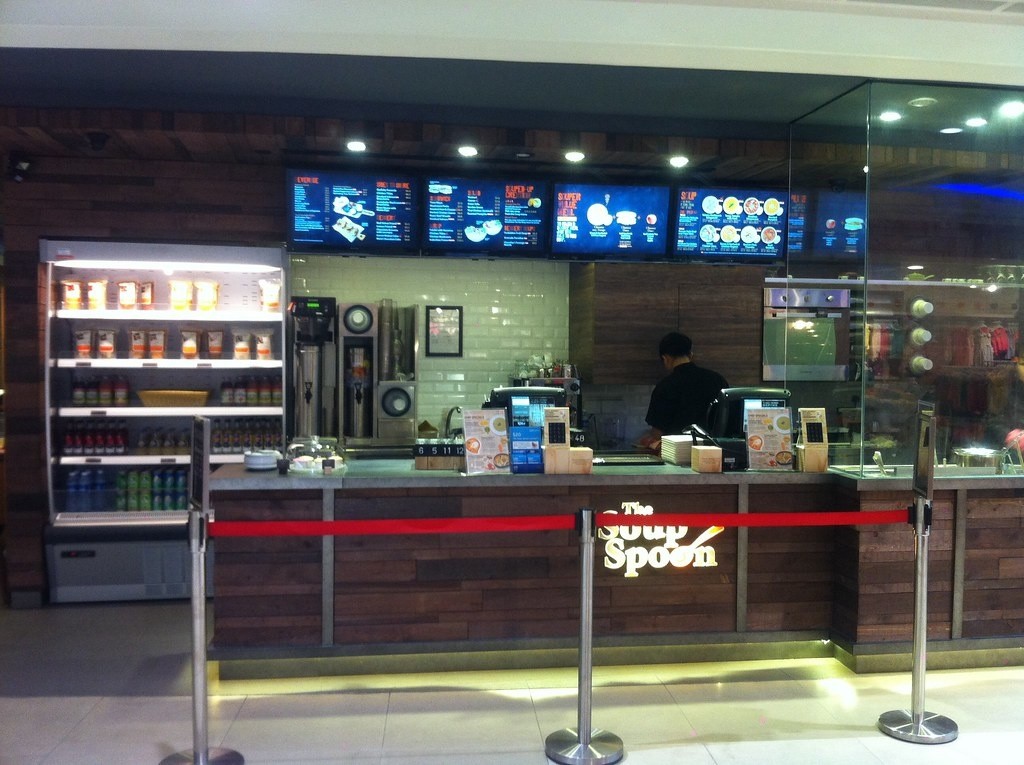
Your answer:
[64, 468, 189, 511]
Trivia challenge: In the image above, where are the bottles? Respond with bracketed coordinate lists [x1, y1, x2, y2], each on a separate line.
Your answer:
[94, 470, 106, 512]
[79, 471, 93, 512]
[177, 429, 190, 454]
[163, 428, 176, 454]
[96, 421, 105, 455]
[72, 375, 131, 407]
[66, 470, 79, 512]
[74, 420, 84, 456]
[84, 421, 96, 456]
[105, 421, 116, 455]
[149, 427, 162, 455]
[211, 418, 282, 453]
[220, 376, 282, 406]
[63, 421, 74, 456]
[115, 419, 129, 455]
[137, 427, 149, 455]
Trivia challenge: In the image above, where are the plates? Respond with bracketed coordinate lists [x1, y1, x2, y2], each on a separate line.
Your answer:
[334, 202, 363, 218]
[587, 204, 608, 226]
[723, 197, 738, 215]
[720, 226, 736, 242]
[748, 435, 764, 451]
[333, 216, 364, 243]
[743, 198, 760, 215]
[490, 415, 507, 436]
[741, 226, 756, 243]
[661, 434, 704, 464]
[700, 225, 716, 242]
[702, 196, 718, 214]
[465, 438, 481, 455]
[764, 198, 779, 215]
[243, 451, 283, 469]
[761, 227, 777, 244]
[773, 414, 790, 434]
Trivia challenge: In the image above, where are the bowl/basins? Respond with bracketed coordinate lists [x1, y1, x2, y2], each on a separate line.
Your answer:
[483, 223, 502, 235]
[136, 388, 212, 406]
[465, 228, 487, 242]
[494, 454, 510, 467]
[775, 452, 792, 465]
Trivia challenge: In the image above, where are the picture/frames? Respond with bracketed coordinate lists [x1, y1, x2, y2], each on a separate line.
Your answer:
[426, 305, 463, 357]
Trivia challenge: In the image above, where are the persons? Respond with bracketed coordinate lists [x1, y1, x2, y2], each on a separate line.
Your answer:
[985, 341, 1024, 464]
[640, 331, 729, 451]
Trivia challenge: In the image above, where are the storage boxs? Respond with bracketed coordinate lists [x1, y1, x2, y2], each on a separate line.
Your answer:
[442, 444, 454, 470]
[454, 444, 466, 470]
[954, 447, 1012, 467]
[570, 431, 586, 447]
[570, 447, 593, 475]
[414, 444, 428, 470]
[691, 446, 723, 473]
[428, 444, 442, 470]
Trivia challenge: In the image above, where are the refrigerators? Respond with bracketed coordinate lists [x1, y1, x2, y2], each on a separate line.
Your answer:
[37, 236, 296, 606]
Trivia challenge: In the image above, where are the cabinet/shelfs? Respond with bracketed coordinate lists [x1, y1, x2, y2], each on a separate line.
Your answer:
[37, 236, 295, 601]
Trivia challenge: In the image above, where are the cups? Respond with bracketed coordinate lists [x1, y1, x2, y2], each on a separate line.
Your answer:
[314, 458, 326, 469]
[277, 459, 289, 474]
[329, 457, 343, 468]
[292, 458, 313, 468]
[379, 299, 404, 380]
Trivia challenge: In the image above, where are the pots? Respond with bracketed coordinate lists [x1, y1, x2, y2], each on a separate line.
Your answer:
[955, 448, 1005, 474]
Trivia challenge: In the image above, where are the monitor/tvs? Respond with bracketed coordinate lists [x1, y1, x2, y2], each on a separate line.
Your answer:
[490, 387, 567, 429]
[712, 386, 791, 438]
